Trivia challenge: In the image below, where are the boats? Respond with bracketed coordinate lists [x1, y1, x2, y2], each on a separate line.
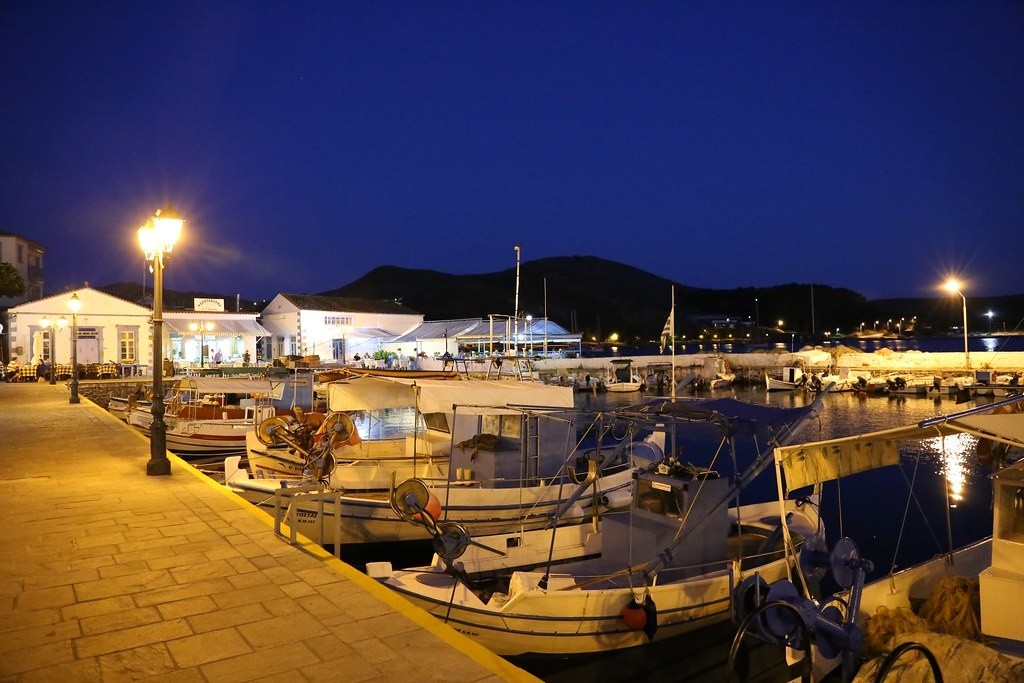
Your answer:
[109, 286, 1024, 683]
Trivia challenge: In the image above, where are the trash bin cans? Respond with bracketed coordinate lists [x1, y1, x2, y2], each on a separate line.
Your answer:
[164, 360, 174, 376]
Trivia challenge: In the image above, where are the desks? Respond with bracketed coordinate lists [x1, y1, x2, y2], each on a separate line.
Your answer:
[56, 365, 72, 381]
[97, 364, 117, 380]
[18, 365, 37, 382]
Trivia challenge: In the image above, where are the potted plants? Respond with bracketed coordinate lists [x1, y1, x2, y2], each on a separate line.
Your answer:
[374, 350, 388, 369]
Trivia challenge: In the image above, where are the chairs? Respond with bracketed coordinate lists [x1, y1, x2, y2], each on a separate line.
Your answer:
[3, 363, 97, 380]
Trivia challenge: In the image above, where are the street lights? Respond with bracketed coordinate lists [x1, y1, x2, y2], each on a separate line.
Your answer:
[137, 209, 187, 478]
[899, 317, 905, 335]
[944, 279, 968, 359]
[859, 322, 865, 333]
[40, 316, 68, 386]
[188, 320, 214, 369]
[66, 292, 81, 403]
[988, 311, 993, 335]
[874, 320, 879, 331]
[525, 315, 533, 356]
[887, 318, 892, 331]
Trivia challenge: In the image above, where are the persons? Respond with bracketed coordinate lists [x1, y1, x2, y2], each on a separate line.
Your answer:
[241, 350, 252, 367]
[0, 355, 73, 383]
[351, 347, 561, 370]
[208, 348, 223, 367]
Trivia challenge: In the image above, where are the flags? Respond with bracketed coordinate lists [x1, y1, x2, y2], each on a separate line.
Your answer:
[659, 308, 673, 353]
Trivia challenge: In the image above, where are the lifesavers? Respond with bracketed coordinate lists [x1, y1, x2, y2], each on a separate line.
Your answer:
[145, 387, 160, 403]
[172, 381, 186, 395]
[975, 402, 1021, 467]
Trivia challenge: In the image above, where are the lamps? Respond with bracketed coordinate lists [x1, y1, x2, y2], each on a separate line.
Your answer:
[41, 316, 67, 334]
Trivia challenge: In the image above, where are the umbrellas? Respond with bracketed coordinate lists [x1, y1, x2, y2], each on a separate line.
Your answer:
[31, 331, 44, 366]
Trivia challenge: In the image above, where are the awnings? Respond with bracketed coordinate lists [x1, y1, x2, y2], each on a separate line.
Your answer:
[163, 319, 270, 336]
